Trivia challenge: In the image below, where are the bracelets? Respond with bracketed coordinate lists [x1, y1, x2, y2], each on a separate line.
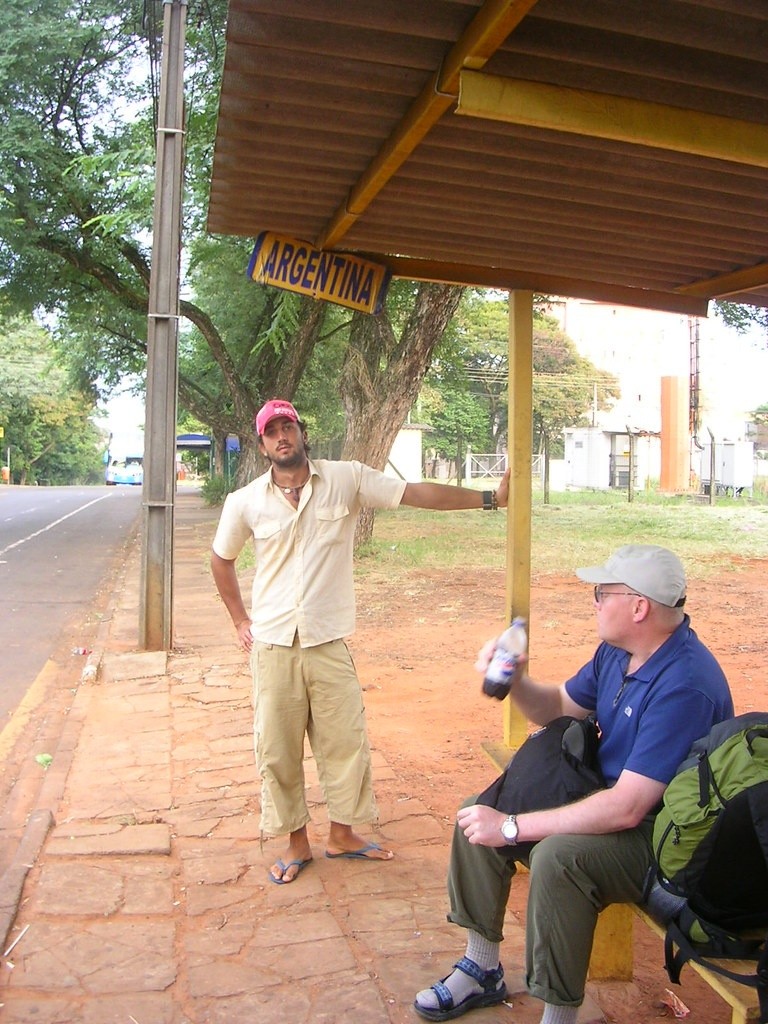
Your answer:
[483, 490, 498, 511]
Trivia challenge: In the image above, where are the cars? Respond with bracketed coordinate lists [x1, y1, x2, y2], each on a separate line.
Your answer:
[106, 454, 143, 486]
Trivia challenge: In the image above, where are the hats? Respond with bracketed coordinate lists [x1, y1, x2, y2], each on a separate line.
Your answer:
[256, 400, 300, 436]
[576, 545, 687, 607]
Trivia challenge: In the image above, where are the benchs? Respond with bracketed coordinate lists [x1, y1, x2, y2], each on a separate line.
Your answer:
[483, 731, 768, 1024]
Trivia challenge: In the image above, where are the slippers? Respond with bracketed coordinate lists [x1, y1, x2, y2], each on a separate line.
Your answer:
[326, 841, 394, 860]
[268, 856, 313, 884]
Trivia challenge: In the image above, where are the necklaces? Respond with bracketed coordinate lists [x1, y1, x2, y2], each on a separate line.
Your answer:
[273, 474, 310, 502]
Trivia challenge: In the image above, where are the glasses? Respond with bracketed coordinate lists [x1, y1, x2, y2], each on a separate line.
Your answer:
[594, 586, 641, 603]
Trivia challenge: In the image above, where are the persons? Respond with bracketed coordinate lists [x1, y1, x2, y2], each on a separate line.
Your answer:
[413, 544, 734, 1024]
[210, 400, 510, 884]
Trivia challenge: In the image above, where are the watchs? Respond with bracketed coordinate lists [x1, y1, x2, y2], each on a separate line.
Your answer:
[501, 814, 518, 846]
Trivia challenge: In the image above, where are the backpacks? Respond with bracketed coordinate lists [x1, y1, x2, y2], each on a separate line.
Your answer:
[474, 711, 607, 861]
[643, 712, 768, 989]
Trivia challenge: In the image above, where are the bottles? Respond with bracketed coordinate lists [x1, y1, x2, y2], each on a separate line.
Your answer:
[482, 616, 527, 698]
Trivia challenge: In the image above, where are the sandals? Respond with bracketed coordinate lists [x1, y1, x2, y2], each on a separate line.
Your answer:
[414, 955, 507, 1021]
